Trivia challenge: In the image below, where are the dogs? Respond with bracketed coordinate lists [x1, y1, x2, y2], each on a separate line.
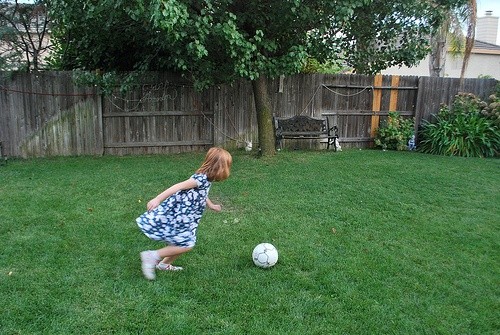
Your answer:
[245, 142, 252, 151]
[335, 137, 342, 152]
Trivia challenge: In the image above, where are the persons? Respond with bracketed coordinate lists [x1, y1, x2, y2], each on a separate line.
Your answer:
[135, 147, 233, 280]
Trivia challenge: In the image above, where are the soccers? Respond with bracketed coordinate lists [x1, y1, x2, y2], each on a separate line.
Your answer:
[252, 243, 278, 268]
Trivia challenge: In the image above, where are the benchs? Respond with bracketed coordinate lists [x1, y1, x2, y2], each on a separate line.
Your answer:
[273, 114, 338, 152]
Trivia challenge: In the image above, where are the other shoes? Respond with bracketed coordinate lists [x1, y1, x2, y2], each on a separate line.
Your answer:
[156, 262, 182, 270]
[140, 250, 157, 280]
